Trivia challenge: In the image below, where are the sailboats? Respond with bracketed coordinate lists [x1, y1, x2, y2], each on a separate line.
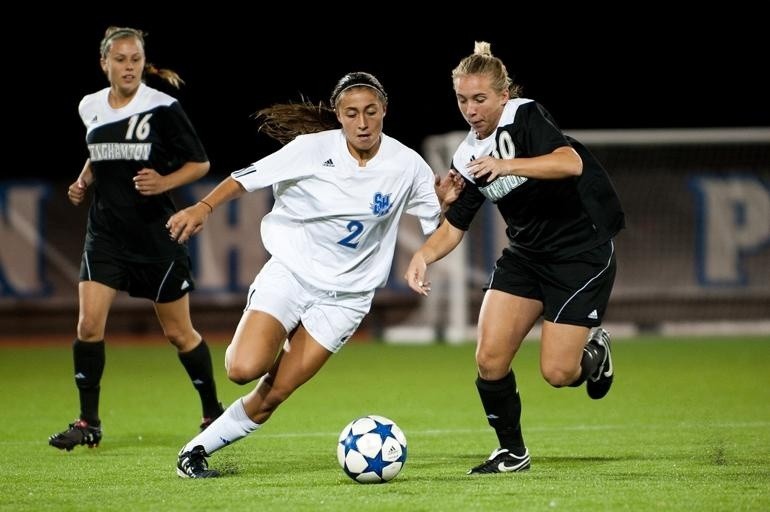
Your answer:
[335, 416, 407, 483]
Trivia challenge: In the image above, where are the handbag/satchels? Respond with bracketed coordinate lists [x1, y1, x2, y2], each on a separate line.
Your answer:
[196, 198, 213, 212]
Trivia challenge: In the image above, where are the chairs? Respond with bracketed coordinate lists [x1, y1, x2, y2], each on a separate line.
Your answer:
[586, 328, 614, 399]
[467, 447, 530, 474]
[49, 419, 102, 451]
[178, 445, 218, 478]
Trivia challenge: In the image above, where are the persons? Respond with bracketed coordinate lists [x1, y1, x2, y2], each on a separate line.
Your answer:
[43, 26, 228, 452]
[163, 70, 468, 479]
[400, 42, 628, 478]
